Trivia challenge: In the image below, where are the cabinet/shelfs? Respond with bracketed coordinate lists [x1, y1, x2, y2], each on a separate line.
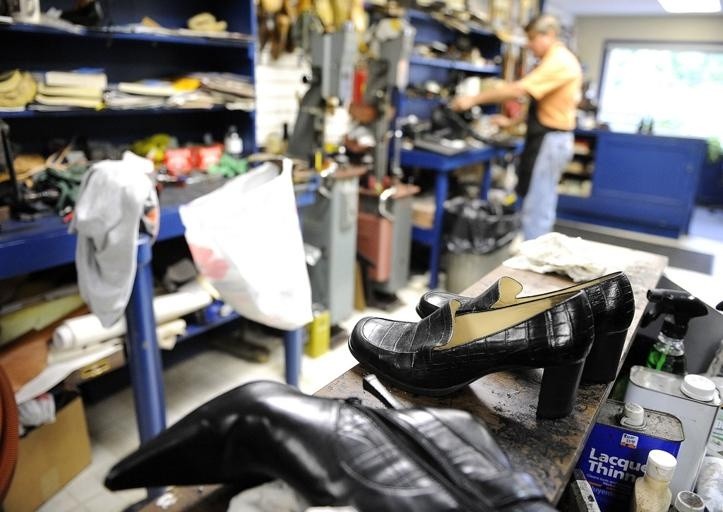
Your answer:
[0, 0, 257, 377]
[550, 136, 599, 219]
[365, 0, 529, 291]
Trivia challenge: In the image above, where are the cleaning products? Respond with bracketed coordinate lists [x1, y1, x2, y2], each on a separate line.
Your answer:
[610, 286, 708, 400]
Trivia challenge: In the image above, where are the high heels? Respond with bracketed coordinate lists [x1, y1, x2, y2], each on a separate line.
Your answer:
[348, 289, 595, 419]
[417, 274, 636, 385]
[103, 370, 556, 512]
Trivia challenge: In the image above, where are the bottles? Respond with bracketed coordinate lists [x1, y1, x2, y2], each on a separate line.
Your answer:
[672, 491, 705, 512]
[634, 449, 677, 512]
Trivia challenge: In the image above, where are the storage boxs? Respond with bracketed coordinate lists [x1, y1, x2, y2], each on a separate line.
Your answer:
[0, 392, 93, 511]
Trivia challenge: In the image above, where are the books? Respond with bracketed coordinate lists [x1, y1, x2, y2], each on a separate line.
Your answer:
[1, 57, 254, 112]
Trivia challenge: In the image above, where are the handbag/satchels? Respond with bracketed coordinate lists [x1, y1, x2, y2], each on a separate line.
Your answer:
[178, 152, 314, 334]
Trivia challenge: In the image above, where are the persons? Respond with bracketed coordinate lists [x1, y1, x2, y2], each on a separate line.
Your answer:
[450, 14, 584, 241]
